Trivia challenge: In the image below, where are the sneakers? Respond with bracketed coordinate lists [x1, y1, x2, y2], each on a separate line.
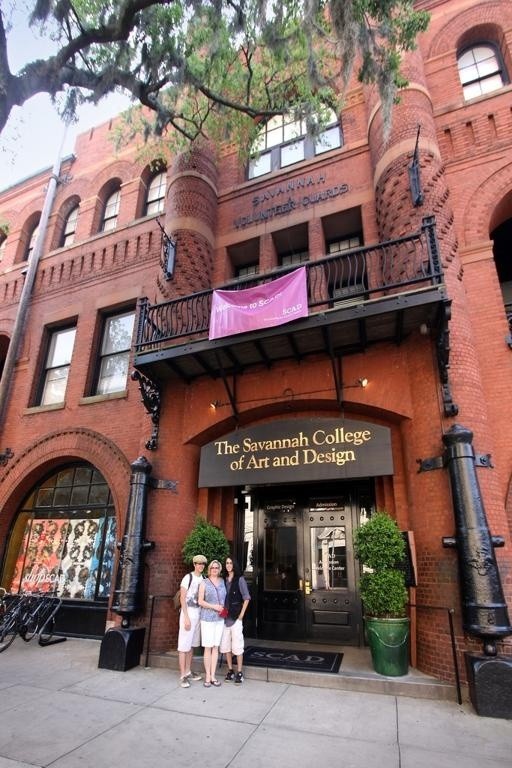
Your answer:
[226, 669, 244, 684]
[180, 671, 202, 687]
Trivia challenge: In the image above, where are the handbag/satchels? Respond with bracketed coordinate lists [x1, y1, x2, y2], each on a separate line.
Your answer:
[218, 608, 228, 618]
[173, 591, 181, 610]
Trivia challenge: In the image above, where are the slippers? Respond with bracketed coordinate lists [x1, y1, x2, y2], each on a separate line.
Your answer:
[204, 679, 220, 687]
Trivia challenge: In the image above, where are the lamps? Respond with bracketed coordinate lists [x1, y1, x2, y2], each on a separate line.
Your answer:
[357, 377, 368, 388]
[210, 398, 218, 408]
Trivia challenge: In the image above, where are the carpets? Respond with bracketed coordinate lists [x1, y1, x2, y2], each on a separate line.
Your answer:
[228, 646, 344, 674]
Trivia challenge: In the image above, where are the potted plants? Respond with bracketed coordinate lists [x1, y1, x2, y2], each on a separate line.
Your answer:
[353, 513, 414, 676]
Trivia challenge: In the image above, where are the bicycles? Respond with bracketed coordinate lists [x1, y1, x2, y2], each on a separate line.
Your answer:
[0, 584, 60, 654]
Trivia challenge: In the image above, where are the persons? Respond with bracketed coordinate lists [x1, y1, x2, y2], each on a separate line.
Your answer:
[197, 559, 227, 688]
[176, 554, 207, 688]
[222, 554, 252, 686]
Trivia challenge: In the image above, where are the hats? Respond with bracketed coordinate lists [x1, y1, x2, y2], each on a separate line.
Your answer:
[193, 555, 208, 563]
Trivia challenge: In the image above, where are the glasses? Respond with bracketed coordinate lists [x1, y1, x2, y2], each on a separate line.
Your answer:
[196, 563, 205, 565]
[211, 567, 218, 569]
